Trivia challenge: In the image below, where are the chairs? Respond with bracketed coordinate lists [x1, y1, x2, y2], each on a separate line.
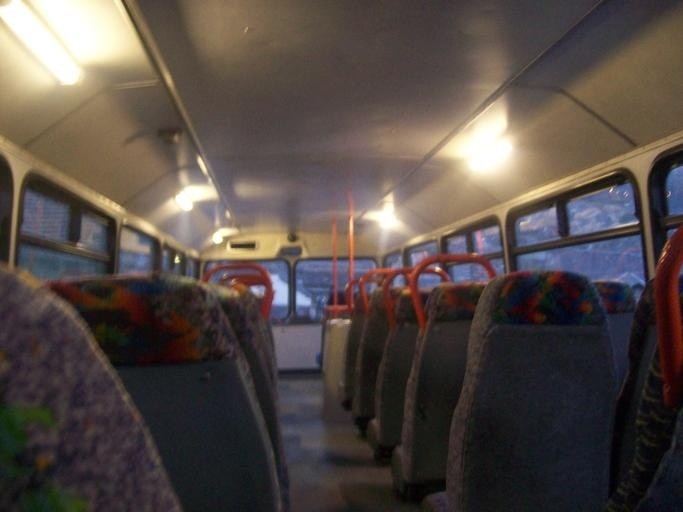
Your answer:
[321, 227, 682, 511]
[2, 228, 298, 511]
